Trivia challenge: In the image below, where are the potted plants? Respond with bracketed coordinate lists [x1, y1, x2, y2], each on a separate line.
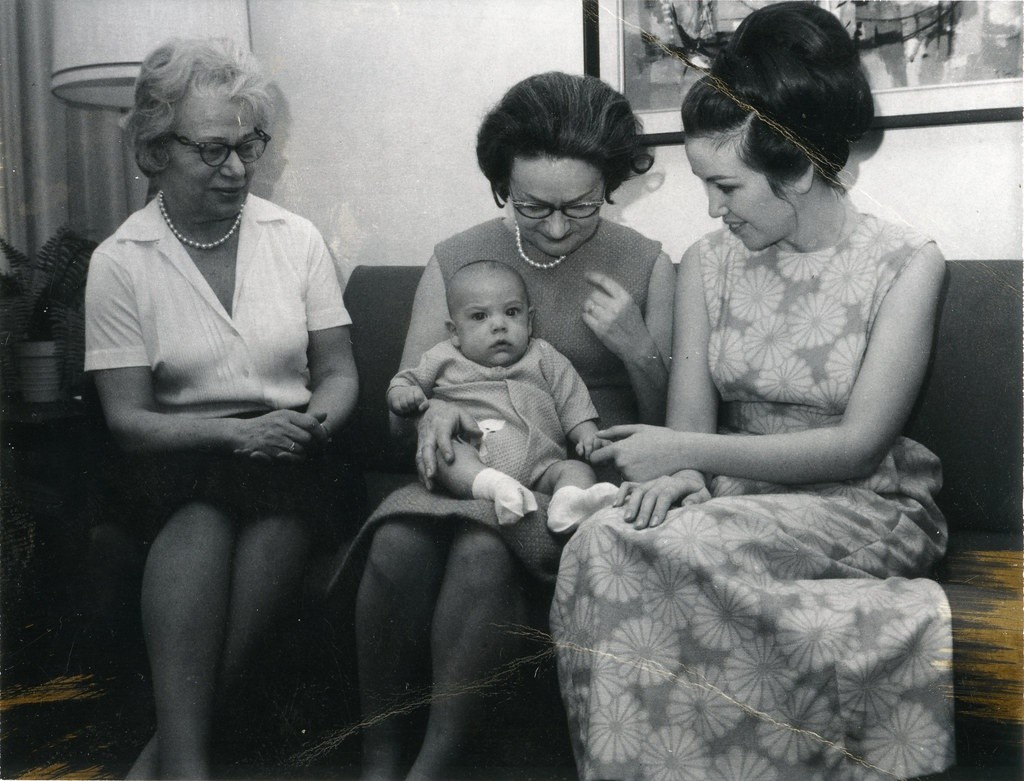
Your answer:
[0, 221, 99, 404]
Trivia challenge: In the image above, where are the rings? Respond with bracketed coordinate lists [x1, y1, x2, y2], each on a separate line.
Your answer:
[289, 442, 296, 452]
[587, 303, 595, 312]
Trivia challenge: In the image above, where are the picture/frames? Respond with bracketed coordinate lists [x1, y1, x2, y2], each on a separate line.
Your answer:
[581, 0, 1024, 147]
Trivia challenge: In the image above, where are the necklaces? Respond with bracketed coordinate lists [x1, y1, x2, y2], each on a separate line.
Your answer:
[158, 190, 246, 249]
[515, 220, 566, 269]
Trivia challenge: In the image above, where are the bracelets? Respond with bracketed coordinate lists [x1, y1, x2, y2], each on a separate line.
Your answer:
[320, 423, 332, 443]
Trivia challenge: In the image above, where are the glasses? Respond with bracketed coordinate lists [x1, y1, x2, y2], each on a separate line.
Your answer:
[506, 181, 608, 219]
[165, 126, 272, 167]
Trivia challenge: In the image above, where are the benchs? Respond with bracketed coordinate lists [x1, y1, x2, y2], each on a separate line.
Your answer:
[295, 259, 1024, 781]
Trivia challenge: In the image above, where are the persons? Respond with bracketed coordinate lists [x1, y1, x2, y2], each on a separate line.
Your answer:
[83, 38, 359, 781]
[387, 260, 626, 534]
[550, 0, 954, 781]
[323, 74, 675, 781]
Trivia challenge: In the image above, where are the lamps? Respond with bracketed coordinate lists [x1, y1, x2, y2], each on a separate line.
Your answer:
[50, 0, 252, 114]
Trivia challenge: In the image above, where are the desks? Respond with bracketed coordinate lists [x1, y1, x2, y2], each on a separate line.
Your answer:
[1, 382, 108, 539]
